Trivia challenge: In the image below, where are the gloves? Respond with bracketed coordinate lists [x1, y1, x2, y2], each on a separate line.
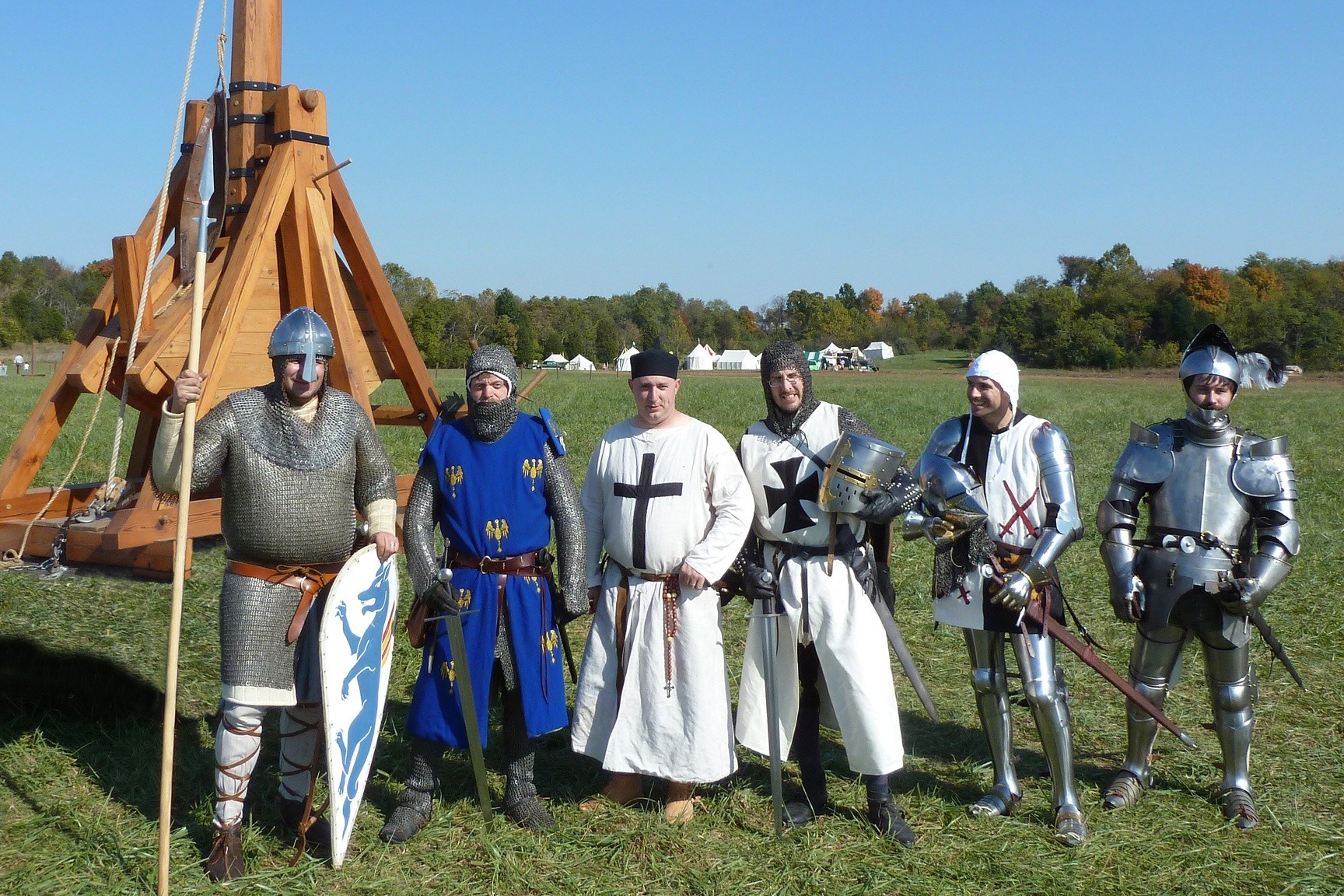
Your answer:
[741, 565, 778, 603]
[853, 489, 903, 527]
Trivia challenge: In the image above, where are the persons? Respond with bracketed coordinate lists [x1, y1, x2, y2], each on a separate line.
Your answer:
[1096, 323, 1300, 827]
[14, 354, 24, 374]
[25, 362, 29, 374]
[902, 349, 1085, 847]
[155, 307, 400, 882]
[380, 344, 589, 842]
[729, 339, 922, 845]
[571, 348, 755, 823]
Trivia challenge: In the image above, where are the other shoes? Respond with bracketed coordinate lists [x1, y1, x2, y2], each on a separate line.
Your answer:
[579, 774, 644, 814]
[206, 820, 244, 883]
[783, 771, 828, 829]
[665, 779, 694, 826]
[865, 788, 915, 845]
[279, 800, 331, 857]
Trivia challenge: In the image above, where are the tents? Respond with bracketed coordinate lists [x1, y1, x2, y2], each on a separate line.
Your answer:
[542, 354, 596, 371]
[717, 350, 762, 370]
[864, 341, 894, 360]
[687, 343, 713, 370]
[803, 342, 842, 368]
[617, 347, 640, 372]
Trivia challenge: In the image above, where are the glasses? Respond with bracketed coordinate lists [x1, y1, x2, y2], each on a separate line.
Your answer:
[766, 373, 803, 387]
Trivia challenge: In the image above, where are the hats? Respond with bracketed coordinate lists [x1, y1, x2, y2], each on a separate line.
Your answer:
[960, 351, 1020, 483]
[629, 337, 680, 381]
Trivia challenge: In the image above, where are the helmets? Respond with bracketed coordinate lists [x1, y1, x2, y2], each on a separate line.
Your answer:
[1178, 324, 1249, 425]
[270, 305, 335, 382]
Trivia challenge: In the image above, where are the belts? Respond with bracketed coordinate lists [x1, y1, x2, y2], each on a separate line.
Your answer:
[443, 549, 554, 646]
[758, 537, 853, 647]
[225, 556, 355, 643]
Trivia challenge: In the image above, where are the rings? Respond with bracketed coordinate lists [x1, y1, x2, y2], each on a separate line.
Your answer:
[391, 543, 395, 546]
